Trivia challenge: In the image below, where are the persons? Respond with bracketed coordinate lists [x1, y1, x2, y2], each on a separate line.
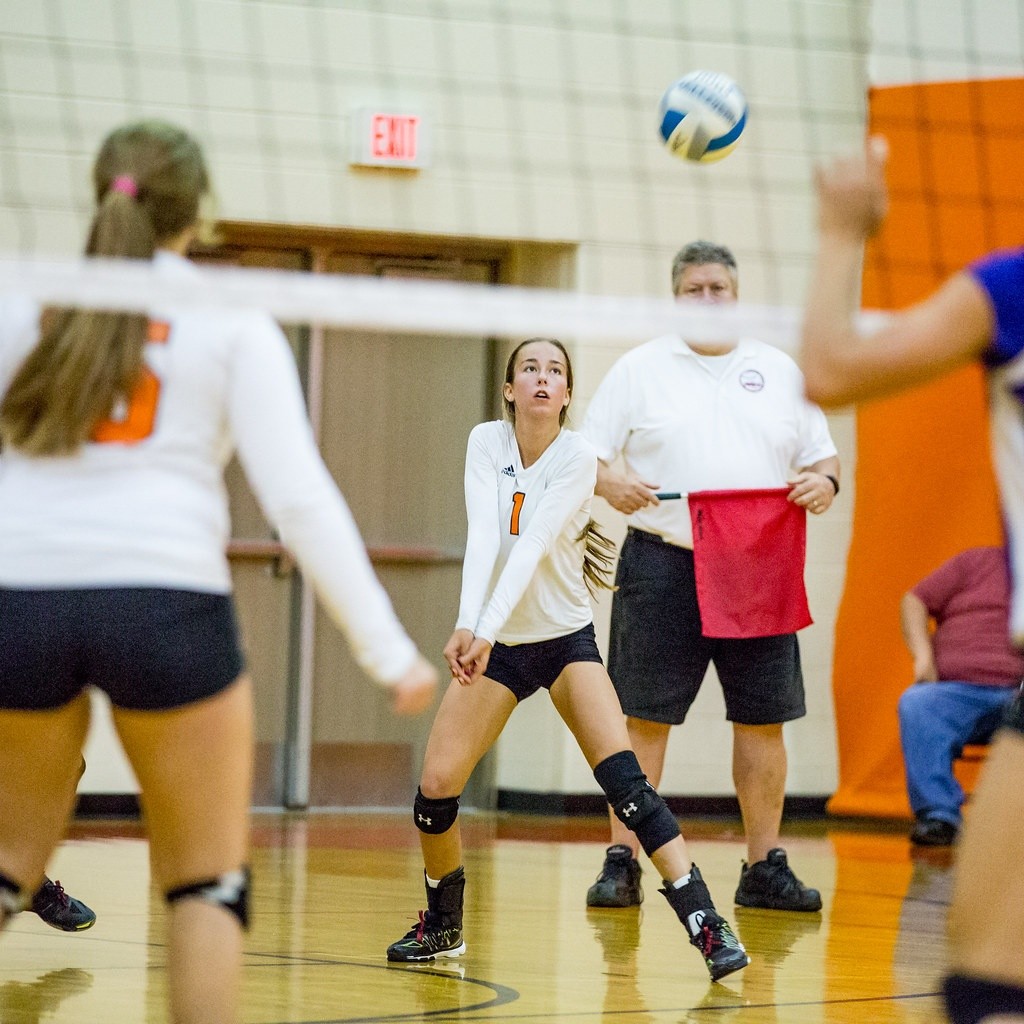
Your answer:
[896, 547, 1024, 846]
[798, 122, 1024, 1024]
[576, 241, 841, 913]
[384, 336, 750, 982]
[0, 120, 441, 1024]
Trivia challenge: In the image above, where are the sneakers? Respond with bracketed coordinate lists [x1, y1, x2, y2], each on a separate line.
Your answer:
[735, 848, 822, 911]
[26, 879, 96, 931]
[910, 818, 957, 844]
[690, 910, 751, 982]
[387, 909, 467, 961]
[586, 844, 645, 907]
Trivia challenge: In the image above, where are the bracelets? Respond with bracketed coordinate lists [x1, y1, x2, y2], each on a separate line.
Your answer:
[827, 474, 840, 496]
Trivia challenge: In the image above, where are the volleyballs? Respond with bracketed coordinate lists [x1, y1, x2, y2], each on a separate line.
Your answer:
[654, 70, 751, 164]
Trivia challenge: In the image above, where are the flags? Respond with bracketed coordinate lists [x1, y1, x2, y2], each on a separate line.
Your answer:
[686, 487, 815, 639]
